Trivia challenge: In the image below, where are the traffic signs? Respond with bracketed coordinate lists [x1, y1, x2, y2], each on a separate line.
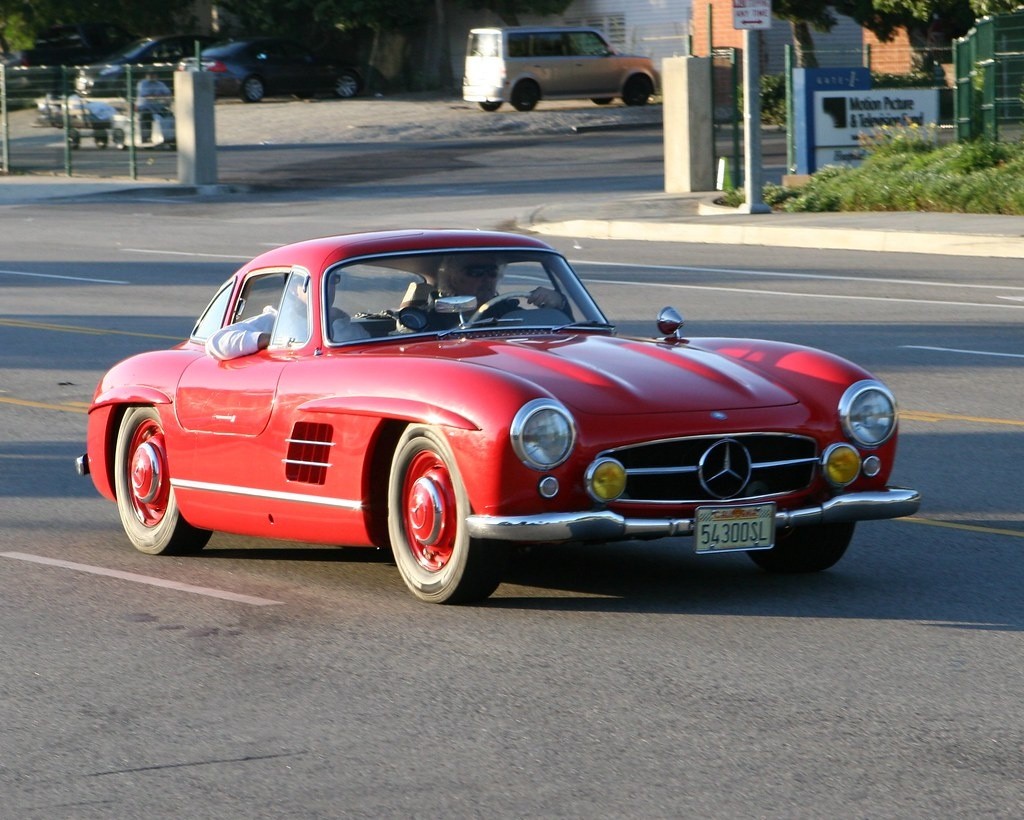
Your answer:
[733, 0, 772, 30]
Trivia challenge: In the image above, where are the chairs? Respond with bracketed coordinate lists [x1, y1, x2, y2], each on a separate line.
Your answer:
[545, 40, 569, 56]
[399, 282, 432, 311]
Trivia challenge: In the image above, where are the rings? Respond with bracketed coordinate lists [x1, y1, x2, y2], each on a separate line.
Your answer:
[540, 302, 546, 308]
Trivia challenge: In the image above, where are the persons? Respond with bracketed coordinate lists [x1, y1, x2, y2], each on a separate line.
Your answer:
[204, 268, 374, 362]
[427, 253, 577, 332]
[39, 64, 171, 145]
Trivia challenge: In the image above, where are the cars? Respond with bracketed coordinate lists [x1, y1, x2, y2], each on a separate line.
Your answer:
[1, 55, 95, 96]
[74, 34, 221, 97]
[177, 37, 365, 103]
[76, 228, 922, 604]
[17, 22, 140, 84]
[462, 26, 662, 112]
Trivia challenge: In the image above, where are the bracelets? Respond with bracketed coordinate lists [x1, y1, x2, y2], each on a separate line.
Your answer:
[557, 292, 566, 310]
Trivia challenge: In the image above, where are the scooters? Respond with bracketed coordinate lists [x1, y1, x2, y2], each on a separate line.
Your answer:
[112, 98, 175, 151]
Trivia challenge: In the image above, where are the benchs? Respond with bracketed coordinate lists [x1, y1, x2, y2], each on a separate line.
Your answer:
[31, 97, 131, 129]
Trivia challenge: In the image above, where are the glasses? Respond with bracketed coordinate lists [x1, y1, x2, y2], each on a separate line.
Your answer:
[460, 263, 499, 278]
[329, 274, 341, 284]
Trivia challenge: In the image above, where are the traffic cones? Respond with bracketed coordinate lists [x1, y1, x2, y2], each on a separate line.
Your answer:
[715, 157, 733, 191]
